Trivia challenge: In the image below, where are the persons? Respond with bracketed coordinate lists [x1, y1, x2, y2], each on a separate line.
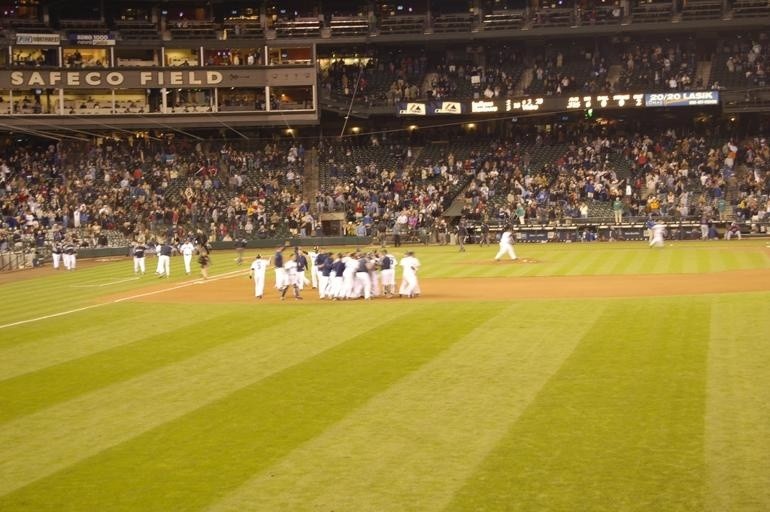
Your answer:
[234, 238, 247, 265]
[250, 245, 421, 300]
[132, 240, 209, 279]
[494, 224, 517, 261]
[0, 0, 770, 255]
[53, 240, 76, 271]
